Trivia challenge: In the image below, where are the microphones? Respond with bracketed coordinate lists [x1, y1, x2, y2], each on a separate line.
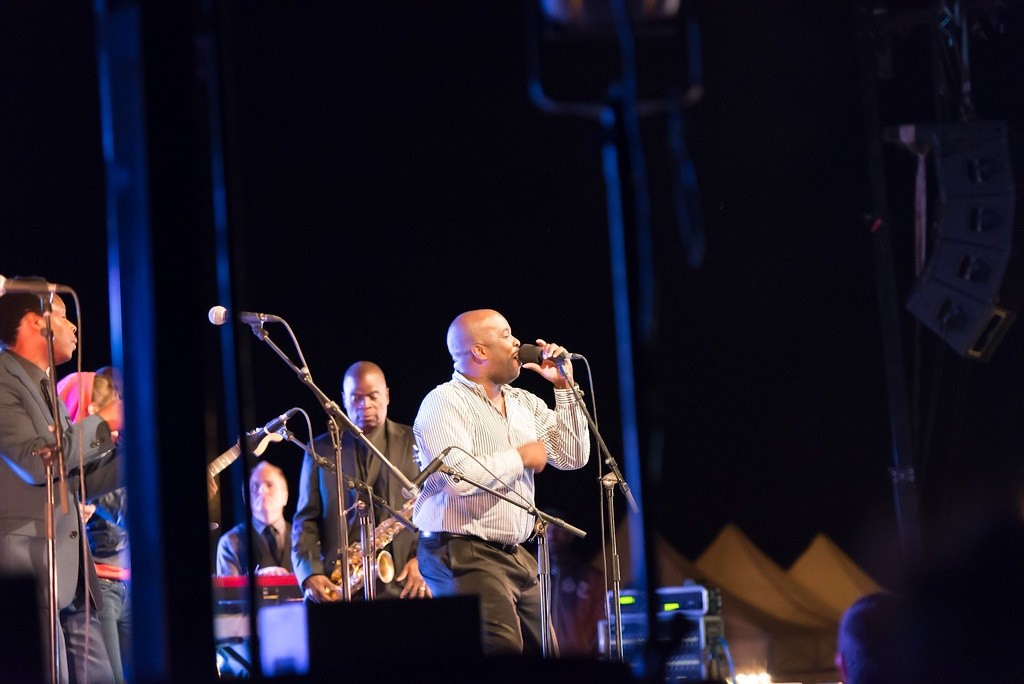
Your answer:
[401, 447, 450, 499]
[517, 344, 582, 364]
[239, 406, 296, 454]
[0, 274, 74, 296]
[209, 306, 281, 325]
[214, 636, 242, 648]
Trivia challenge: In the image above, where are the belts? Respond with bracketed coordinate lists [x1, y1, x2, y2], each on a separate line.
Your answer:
[441, 533, 518, 554]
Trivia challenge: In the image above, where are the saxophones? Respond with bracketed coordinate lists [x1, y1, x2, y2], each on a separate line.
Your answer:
[330, 442, 424, 599]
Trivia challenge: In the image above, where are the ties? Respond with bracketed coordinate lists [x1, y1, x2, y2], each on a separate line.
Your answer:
[265, 525, 281, 567]
[40, 379, 54, 418]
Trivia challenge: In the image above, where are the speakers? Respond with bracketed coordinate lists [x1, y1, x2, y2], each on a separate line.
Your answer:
[211, 596, 308, 678]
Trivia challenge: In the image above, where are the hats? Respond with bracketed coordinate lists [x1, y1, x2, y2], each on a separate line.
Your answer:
[57, 370, 94, 425]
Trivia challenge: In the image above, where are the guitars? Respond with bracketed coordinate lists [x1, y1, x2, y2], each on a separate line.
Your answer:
[208, 424, 283, 479]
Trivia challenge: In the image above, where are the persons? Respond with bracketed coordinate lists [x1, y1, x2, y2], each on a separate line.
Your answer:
[834, 593, 935, 684]
[412, 309, 591, 655]
[289, 361, 432, 601]
[0, 277, 129, 684]
[216, 460, 301, 577]
